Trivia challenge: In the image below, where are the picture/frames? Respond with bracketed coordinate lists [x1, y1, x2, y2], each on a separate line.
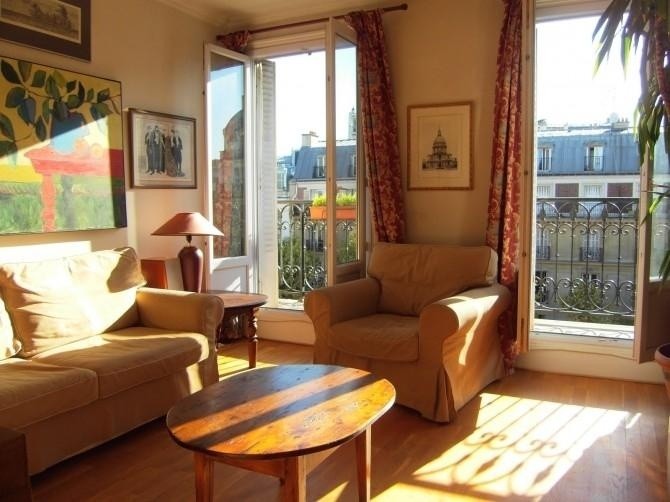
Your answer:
[407, 100, 474, 191]
[0, 0, 91, 63]
[129, 108, 197, 189]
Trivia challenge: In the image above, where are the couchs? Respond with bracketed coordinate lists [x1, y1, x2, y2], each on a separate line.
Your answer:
[0, 287, 225, 502]
[304, 241, 513, 424]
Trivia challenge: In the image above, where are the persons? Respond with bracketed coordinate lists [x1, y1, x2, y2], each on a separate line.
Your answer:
[145, 124, 183, 176]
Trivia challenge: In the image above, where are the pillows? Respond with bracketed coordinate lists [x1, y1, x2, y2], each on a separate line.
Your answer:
[0, 247, 148, 360]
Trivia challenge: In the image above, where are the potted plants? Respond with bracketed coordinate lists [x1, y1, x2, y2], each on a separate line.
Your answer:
[308, 192, 358, 220]
[587, 0, 670, 407]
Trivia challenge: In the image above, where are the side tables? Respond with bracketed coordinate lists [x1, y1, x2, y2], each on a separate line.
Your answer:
[201, 291, 270, 369]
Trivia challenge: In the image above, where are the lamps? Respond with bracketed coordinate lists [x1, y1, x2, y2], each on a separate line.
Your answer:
[150, 211, 226, 294]
